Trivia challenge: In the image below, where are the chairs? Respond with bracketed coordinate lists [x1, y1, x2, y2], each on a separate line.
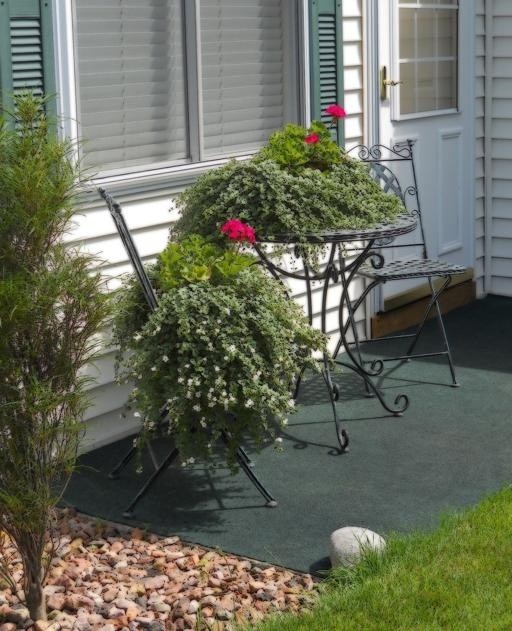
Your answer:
[320, 138, 465, 387]
[97, 187, 281, 515]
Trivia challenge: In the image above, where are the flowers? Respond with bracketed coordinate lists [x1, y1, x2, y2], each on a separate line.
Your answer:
[210, 217, 259, 254]
[297, 103, 346, 148]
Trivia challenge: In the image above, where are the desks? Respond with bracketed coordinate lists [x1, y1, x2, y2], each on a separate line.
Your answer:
[225, 205, 417, 453]
